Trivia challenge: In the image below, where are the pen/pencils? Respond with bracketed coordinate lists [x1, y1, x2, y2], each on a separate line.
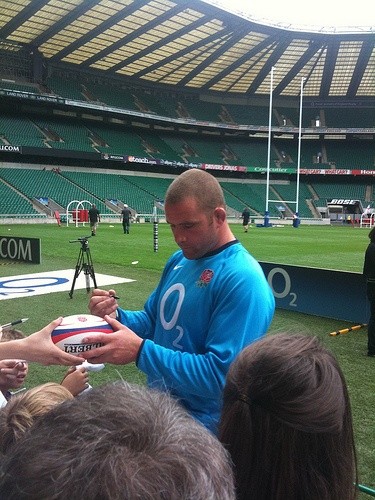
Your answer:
[109, 294, 120, 299]
[1, 317, 30, 329]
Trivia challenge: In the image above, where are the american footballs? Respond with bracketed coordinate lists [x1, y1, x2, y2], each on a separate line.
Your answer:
[51, 313, 115, 357]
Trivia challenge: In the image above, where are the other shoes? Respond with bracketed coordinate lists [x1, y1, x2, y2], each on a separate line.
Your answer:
[124, 231, 129, 234]
[92, 233, 96, 236]
[368, 350, 375, 358]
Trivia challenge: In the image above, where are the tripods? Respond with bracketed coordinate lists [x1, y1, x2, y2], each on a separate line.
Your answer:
[68, 236, 98, 299]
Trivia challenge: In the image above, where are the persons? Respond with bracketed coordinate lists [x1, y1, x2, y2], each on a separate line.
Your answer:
[0, 316, 88, 385]
[82, 167, 274, 452]
[120, 204, 132, 234]
[242, 207, 250, 232]
[0, 328, 30, 409]
[0, 367, 240, 500]
[88, 205, 100, 236]
[363, 225, 375, 356]
[219, 327, 361, 500]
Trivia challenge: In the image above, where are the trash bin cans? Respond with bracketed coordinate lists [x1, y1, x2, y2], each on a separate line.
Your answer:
[73, 209, 89, 222]
